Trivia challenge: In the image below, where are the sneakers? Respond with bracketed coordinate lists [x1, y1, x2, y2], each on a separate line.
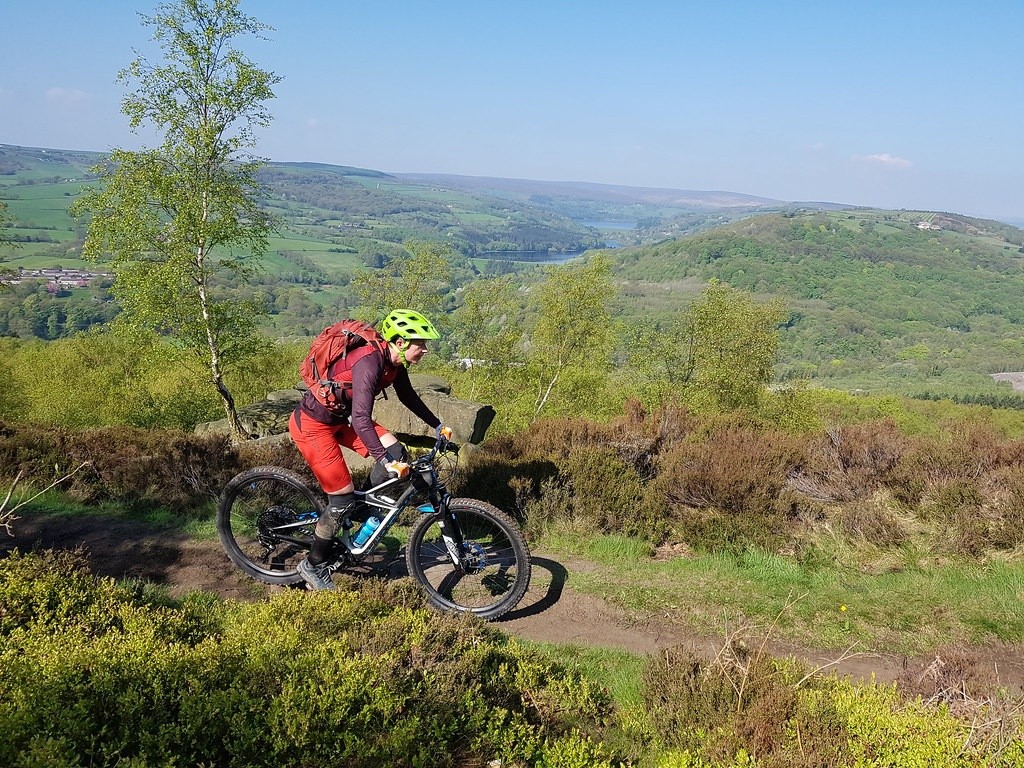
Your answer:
[296, 557, 336, 590]
[349, 503, 385, 523]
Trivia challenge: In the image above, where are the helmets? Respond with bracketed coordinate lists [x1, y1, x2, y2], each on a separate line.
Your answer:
[382, 308, 441, 341]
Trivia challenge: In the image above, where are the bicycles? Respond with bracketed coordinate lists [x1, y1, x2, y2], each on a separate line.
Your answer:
[215, 435, 531, 621]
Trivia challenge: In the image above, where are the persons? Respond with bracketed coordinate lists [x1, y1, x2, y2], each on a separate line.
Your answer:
[289, 309, 452, 593]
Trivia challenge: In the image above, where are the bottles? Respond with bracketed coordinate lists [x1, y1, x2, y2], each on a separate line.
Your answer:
[353, 516, 380, 545]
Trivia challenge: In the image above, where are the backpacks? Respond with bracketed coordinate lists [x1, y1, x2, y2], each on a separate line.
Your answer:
[299, 319, 398, 415]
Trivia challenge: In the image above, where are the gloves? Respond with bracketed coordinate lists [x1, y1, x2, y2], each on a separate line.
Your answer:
[386, 459, 411, 479]
[439, 426, 453, 441]
